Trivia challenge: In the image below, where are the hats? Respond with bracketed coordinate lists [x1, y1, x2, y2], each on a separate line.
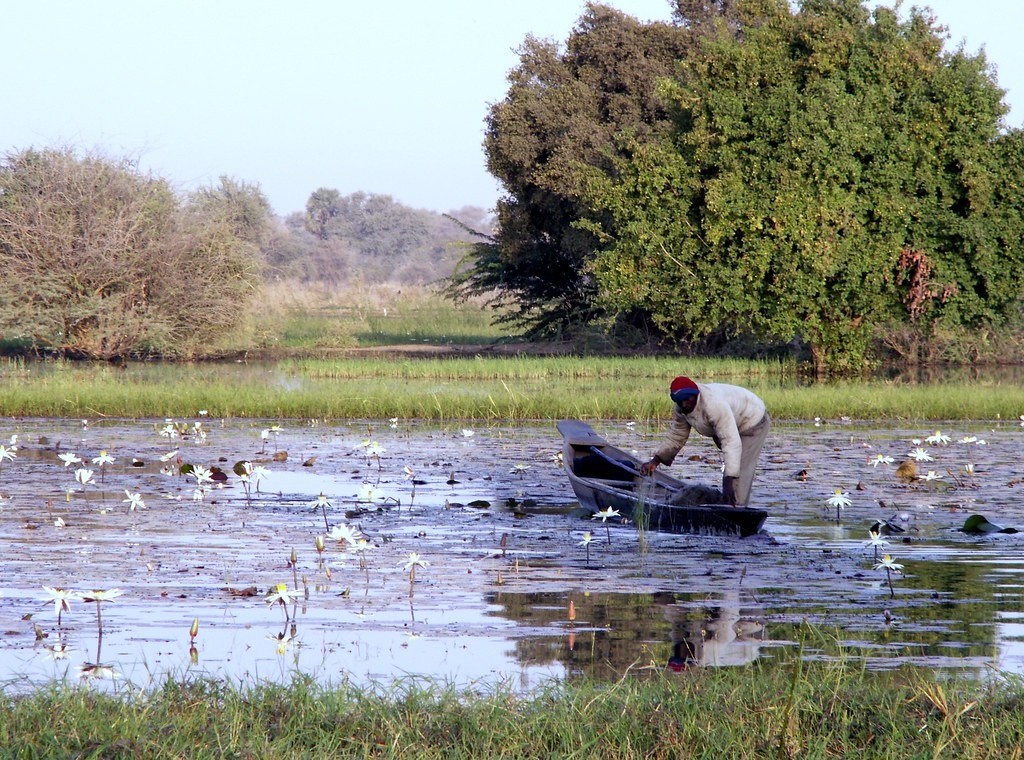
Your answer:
[670, 376, 700, 402]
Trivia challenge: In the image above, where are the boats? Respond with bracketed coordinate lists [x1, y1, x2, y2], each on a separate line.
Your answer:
[555, 417, 769, 540]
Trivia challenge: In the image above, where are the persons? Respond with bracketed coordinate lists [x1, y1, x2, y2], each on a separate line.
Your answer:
[652, 586, 764, 675]
[639, 376, 770, 507]
